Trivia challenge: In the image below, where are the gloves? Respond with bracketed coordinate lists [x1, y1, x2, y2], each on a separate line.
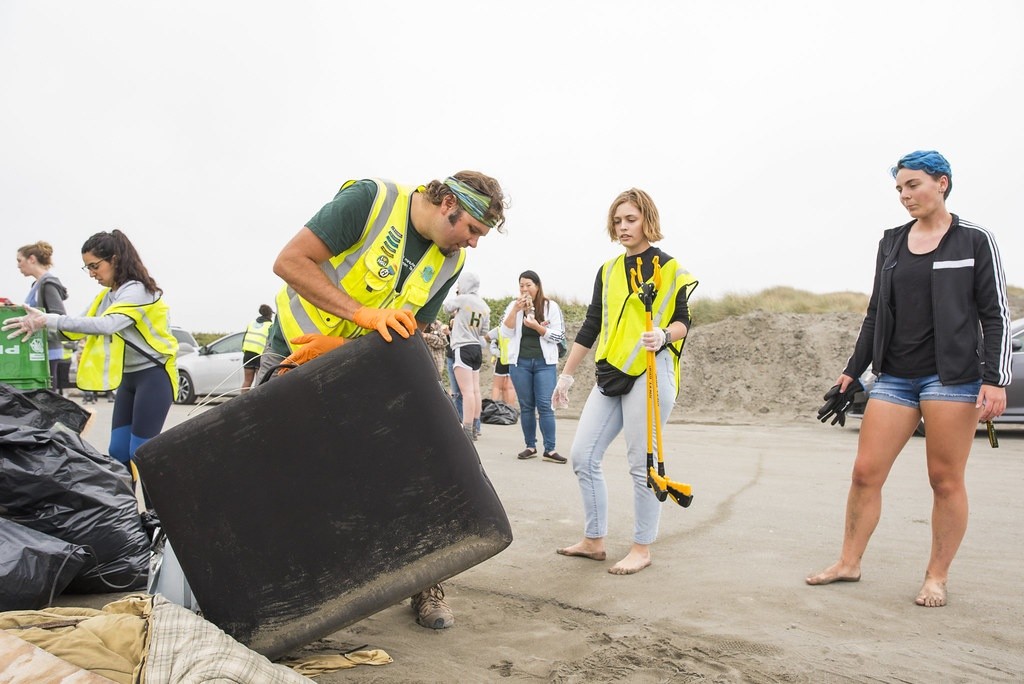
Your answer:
[817, 378, 865, 427]
[353, 304, 419, 342]
[276, 333, 345, 375]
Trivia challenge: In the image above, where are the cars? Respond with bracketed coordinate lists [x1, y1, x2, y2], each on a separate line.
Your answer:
[174, 331, 256, 404]
[912, 317, 1024, 437]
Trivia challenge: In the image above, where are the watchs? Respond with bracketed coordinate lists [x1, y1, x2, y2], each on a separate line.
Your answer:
[661, 328, 672, 347]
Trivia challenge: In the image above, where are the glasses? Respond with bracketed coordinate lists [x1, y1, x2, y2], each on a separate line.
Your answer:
[81, 257, 110, 273]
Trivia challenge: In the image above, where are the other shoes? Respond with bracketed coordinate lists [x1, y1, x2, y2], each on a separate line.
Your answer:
[517, 447, 538, 459]
[542, 451, 567, 464]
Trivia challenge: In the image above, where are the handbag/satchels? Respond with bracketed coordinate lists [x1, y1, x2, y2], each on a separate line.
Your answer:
[479, 398, 518, 425]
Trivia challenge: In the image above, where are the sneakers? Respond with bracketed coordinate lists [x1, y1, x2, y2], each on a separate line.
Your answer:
[411, 584, 454, 629]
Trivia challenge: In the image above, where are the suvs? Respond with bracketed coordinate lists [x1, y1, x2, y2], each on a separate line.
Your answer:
[170, 326, 200, 358]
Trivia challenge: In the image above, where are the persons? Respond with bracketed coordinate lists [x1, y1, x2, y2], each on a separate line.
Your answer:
[501, 270, 568, 464]
[1, 228, 179, 512]
[0, 242, 69, 391]
[550, 189, 700, 576]
[803, 150, 1012, 609]
[441, 272, 492, 442]
[254, 169, 506, 389]
[485, 315, 516, 405]
[241, 303, 275, 393]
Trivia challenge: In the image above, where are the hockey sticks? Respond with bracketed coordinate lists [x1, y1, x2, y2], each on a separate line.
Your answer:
[629, 254, 694, 509]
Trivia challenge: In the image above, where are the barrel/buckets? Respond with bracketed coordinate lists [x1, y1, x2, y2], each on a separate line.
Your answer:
[0, 305, 53, 391]
